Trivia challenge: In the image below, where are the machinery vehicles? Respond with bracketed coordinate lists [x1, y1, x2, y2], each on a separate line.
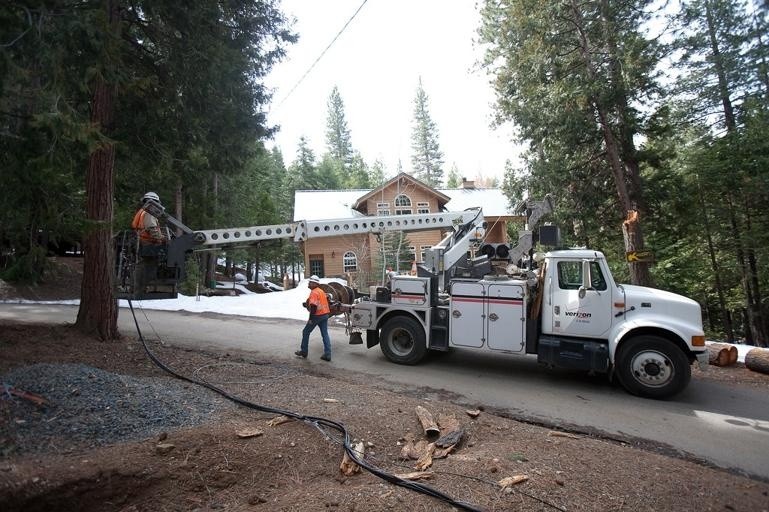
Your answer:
[108, 202, 710, 402]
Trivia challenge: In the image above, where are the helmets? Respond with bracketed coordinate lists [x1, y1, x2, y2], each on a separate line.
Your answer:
[143, 192, 159, 204]
[308, 273, 319, 282]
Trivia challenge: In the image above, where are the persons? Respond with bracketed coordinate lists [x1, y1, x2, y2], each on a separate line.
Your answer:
[294, 274, 332, 361]
[132, 192, 169, 248]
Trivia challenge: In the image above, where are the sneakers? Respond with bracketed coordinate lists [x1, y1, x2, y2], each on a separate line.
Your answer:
[295, 350, 307, 358]
[319, 354, 330, 360]
